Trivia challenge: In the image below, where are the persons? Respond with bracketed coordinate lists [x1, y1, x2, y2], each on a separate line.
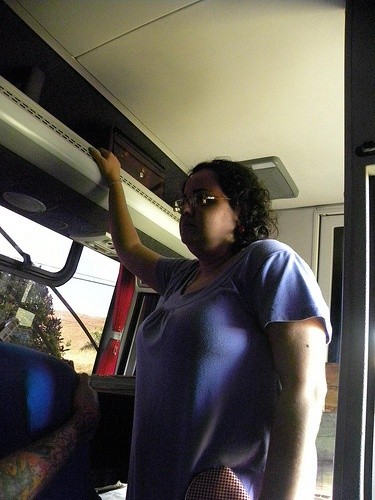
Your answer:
[88, 147, 332, 500]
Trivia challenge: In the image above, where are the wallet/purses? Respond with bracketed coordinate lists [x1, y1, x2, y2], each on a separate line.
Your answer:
[185, 466, 250, 500]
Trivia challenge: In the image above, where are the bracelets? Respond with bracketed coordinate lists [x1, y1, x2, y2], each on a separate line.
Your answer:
[105, 178, 123, 187]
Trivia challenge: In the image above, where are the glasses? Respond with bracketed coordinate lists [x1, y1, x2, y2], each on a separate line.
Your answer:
[173, 195, 231, 212]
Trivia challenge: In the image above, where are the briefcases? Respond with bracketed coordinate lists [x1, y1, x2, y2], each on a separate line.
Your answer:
[110, 125, 166, 198]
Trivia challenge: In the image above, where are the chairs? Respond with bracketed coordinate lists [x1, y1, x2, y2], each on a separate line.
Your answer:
[0, 342, 101, 500]
